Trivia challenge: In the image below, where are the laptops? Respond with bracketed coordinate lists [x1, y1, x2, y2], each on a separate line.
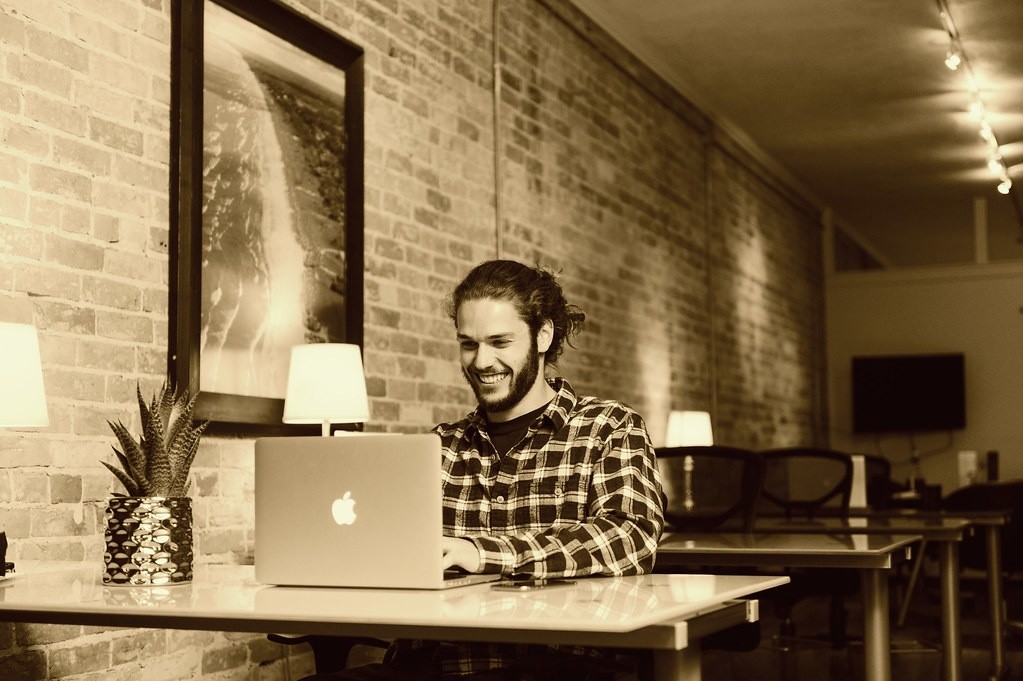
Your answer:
[255, 432, 502, 589]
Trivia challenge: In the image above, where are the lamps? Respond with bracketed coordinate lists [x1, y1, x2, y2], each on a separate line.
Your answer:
[849, 454, 867, 511]
[937, 0, 1013, 200]
[664, 410, 713, 511]
[280, 342, 369, 436]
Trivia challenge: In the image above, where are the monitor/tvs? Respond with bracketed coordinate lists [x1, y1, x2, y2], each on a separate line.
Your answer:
[852, 352, 966, 436]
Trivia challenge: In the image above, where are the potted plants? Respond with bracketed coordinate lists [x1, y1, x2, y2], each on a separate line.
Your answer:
[100, 371, 212, 588]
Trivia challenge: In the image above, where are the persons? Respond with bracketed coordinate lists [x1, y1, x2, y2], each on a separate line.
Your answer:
[295, 259, 668, 681]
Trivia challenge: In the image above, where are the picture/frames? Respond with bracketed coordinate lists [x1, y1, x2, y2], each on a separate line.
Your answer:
[167, 0, 367, 436]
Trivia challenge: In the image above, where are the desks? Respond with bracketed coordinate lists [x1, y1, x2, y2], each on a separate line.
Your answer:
[658, 529, 923, 681]
[661, 506, 979, 681]
[0, 561, 791, 681]
[851, 505, 1015, 681]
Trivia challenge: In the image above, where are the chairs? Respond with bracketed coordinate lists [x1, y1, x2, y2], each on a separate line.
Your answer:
[655, 446, 766, 646]
[755, 447, 853, 649]
